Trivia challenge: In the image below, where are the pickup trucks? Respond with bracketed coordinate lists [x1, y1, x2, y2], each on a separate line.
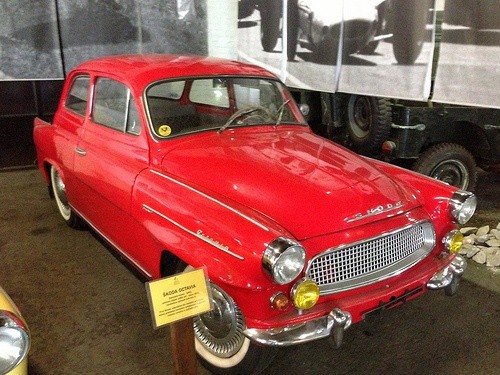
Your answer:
[29, 56, 478, 375]
